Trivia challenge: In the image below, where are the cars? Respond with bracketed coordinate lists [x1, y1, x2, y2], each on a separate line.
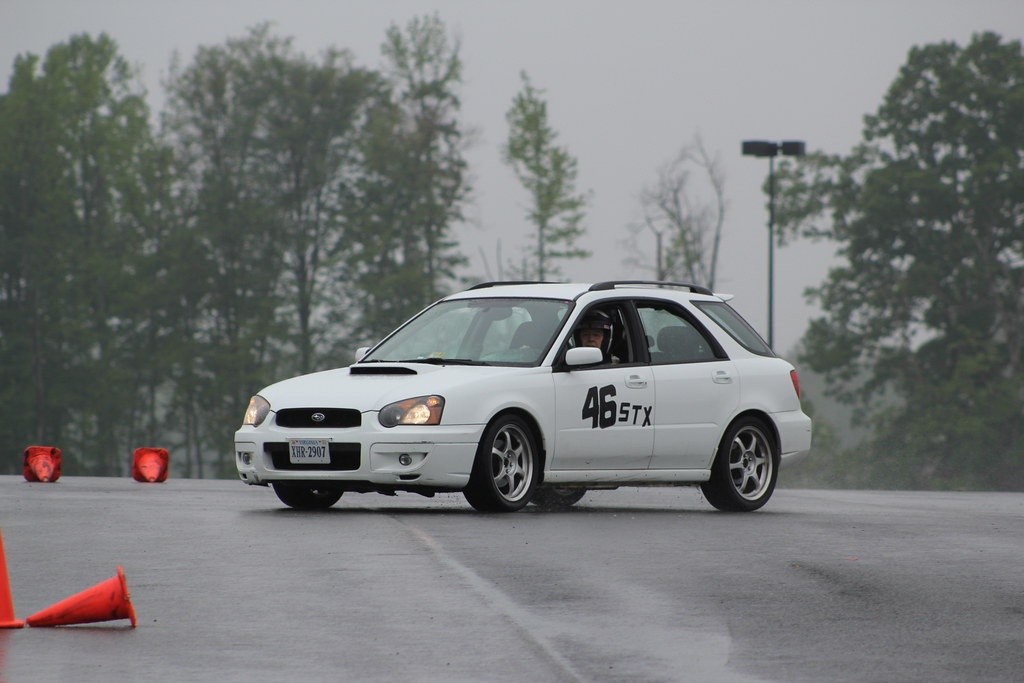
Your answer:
[233, 279, 813, 515]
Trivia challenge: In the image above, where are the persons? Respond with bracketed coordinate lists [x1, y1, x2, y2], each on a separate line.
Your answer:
[570, 308, 621, 368]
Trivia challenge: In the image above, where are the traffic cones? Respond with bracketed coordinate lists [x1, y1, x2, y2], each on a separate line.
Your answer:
[24, 445, 62, 483]
[131, 447, 168, 484]
[0, 532, 24, 630]
[25, 566, 138, 629]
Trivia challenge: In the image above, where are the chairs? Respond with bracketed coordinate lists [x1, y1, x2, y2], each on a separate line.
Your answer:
[649, 326, 712, 361]
[510, 319, 554, 350]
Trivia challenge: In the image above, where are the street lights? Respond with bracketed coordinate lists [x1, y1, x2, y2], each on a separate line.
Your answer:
[743, 139, 806, 346]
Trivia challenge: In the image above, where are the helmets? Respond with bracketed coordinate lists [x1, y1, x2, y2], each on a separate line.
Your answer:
[571, 306, 617, 357]
